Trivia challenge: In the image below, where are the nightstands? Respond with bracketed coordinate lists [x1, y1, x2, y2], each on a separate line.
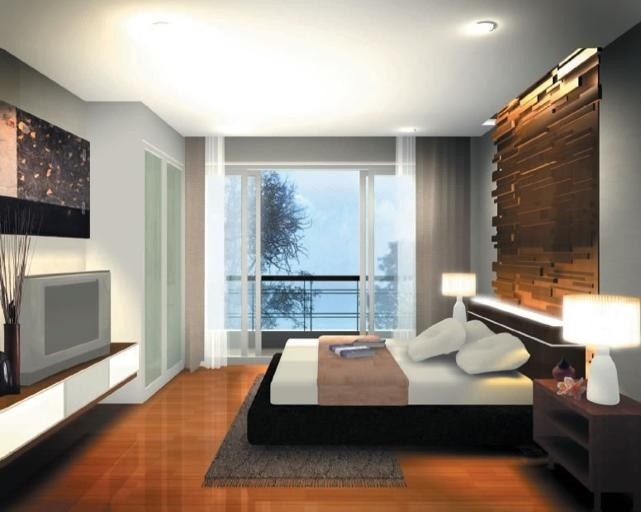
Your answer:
[532, 378, 640, 512]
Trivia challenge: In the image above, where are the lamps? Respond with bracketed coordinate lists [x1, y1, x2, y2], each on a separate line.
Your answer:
[440, 272, 476, 321]
[561, 294, 639, 405]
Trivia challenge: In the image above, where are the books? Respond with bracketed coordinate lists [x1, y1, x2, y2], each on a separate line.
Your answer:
[329, 344, 374, 357]
[353, 339, 385, 348]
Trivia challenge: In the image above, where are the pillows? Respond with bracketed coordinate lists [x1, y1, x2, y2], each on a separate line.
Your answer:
[455, 332, 530, 374]
[464, 319, 495, 345]
[407, 318, 465, 361]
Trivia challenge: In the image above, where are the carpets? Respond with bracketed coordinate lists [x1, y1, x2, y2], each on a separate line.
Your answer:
[199, 372, 406, 487]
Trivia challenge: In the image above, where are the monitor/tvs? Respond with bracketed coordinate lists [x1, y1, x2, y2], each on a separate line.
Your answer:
[15, 270, 111, 386]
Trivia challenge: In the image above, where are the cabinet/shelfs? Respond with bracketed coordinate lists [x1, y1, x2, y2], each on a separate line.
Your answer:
[0, 342, 139, 469]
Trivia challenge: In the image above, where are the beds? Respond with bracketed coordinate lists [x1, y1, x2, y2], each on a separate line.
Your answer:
[246, 298, 585, 446]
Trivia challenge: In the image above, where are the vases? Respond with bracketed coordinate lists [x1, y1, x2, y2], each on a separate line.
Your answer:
[4, 321, 21, 394]
